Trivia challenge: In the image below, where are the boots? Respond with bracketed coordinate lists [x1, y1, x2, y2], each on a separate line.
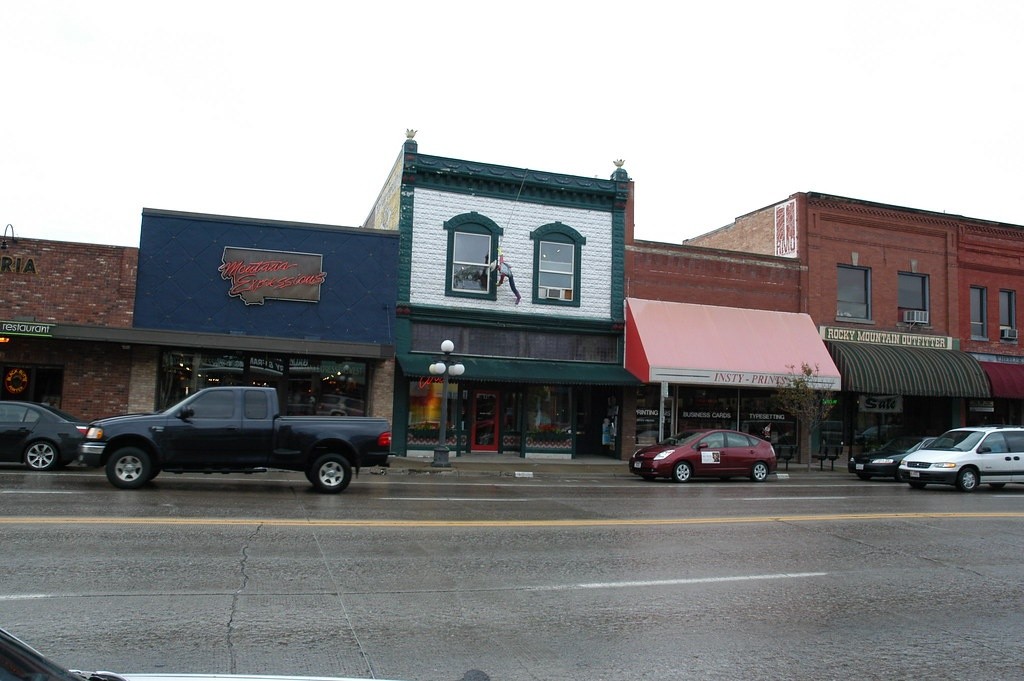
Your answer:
[514, 292, 521, 304]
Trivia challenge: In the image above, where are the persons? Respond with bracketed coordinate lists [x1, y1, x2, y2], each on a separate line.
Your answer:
[491, 248, 521, 304]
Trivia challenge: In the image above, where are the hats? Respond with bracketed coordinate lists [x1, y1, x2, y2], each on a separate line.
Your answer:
[490, 260, 497, 272]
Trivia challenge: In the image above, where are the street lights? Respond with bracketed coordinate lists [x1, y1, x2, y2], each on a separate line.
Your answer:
[428, 340, 464, 468]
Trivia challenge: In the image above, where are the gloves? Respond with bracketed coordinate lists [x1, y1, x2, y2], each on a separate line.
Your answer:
[497, 247, 502, 256]
[495, 281, 502, 286]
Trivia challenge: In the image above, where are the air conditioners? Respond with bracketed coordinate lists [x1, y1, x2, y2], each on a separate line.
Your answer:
[1001, 329, 1018, 339]
[903, 310, 928, 322]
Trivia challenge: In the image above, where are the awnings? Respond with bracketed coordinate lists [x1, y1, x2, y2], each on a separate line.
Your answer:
[399, 355, 642, 387]
[979, 361, 1024, 398]
[826, 341, 990, 398]
[622, 297, 841, 391]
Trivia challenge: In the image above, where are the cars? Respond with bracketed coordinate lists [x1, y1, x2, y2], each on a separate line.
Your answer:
[663, 402, 731, 427]
[782, 421, 843, 446]
[854, 425, 902, 445]
[0, 400, 90, 471]
[317, 394, 365, 416]
[848, 436, 955, 483]
[629, 429, 777, 483]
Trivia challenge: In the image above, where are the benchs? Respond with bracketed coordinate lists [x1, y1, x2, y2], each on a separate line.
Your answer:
[812, 445, 844, 469]
[773, 445, 797, 469]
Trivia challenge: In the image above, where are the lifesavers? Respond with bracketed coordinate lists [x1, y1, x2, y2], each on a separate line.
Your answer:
[6, 368, 27, 394]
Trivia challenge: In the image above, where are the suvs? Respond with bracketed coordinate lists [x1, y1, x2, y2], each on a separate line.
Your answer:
[898, 424, 1024, 493]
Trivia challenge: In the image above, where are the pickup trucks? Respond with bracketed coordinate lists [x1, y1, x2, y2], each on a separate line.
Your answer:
[79, 387, 391, 494]
